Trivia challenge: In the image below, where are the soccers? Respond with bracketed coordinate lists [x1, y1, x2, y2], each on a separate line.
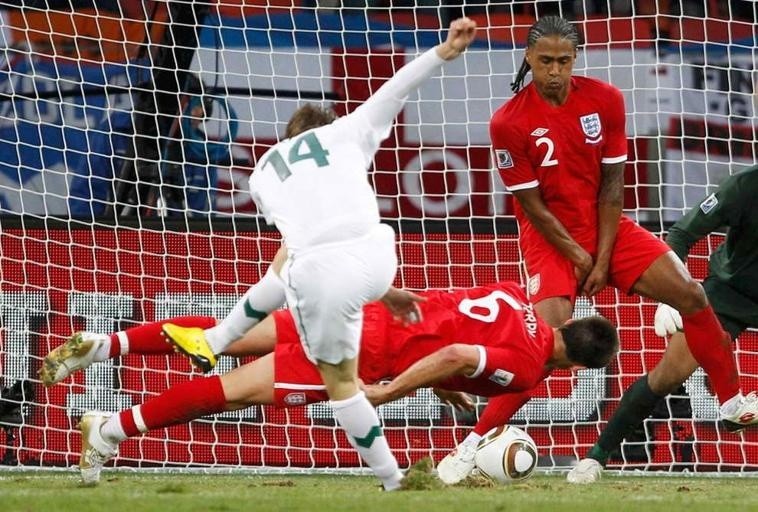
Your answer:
[475, 425, 538, 483]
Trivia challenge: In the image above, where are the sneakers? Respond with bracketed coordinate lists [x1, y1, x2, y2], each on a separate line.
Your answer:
[75, 409, 119, 482]
[437, 440, 478, 486]
[566, 458, 604, 484]
[39, 331, 108, 388]
[160, 322, 218, 373]
[719, 394, 758, 432]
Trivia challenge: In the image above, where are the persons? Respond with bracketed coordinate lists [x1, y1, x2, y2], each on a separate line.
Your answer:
[566, 159, 758, 491]
[37, 280, 623, 489]
[432, 16, 757, 486]
[161, 16, 480, 492]
[61, 54, 221, 234]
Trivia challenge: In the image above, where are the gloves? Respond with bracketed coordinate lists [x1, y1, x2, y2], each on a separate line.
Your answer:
[654, 301, 684, 338]
